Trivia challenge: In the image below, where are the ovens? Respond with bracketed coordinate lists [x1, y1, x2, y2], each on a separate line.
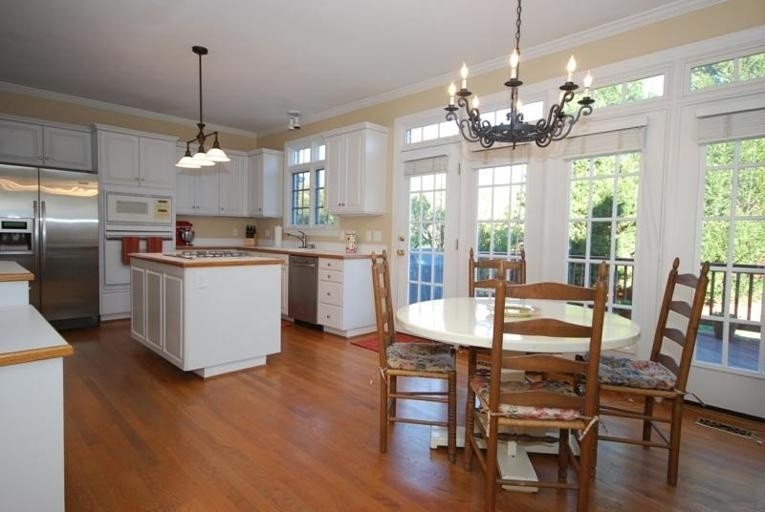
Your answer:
[103, 187, 173, 288]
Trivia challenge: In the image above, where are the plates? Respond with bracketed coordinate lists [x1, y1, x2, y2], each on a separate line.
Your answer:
[486, 302, 543, 317]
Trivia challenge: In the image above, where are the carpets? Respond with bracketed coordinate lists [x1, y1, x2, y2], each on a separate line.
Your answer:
[350, 334, 442, 353]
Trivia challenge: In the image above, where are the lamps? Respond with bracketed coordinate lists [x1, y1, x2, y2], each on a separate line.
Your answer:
[443, 0, 595, 150]
[176, 46, 231, 169]
[287, 110, 301, 131]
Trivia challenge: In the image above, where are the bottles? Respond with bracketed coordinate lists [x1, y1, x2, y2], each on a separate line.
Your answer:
[345, 232, 358, 253]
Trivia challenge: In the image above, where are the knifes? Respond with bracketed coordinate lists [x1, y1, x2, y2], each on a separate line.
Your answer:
[246, 224, 256, 237]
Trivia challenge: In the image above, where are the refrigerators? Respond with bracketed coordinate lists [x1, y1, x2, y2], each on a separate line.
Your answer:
[0, 163, 100, 332]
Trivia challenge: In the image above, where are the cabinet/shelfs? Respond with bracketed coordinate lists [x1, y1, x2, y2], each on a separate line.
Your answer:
[317, 257, 386, 341]
[247, 148, 283, 219]
[176, 141, 220, 217]
[0, 112, 92, 173]
[95, 122, 179, 190]
[221, 148, 249, 218]
[324, 122, 387, 215]
[246, 251, 290, 320]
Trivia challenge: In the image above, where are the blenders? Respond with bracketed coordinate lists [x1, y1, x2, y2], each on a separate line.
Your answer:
[176, 221, 195, 247]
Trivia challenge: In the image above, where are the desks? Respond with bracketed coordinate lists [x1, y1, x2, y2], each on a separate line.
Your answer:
[396, 296, 641, 493]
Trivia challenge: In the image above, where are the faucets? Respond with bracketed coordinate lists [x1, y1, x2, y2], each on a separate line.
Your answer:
[286, 230, 307, 248]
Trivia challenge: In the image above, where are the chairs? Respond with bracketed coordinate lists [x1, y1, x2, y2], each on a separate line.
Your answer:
[465, 261, 609, 510]
[469, 248, 526, 376]
[371, 250, 457, 463]
[571, 257, 710, 486]
